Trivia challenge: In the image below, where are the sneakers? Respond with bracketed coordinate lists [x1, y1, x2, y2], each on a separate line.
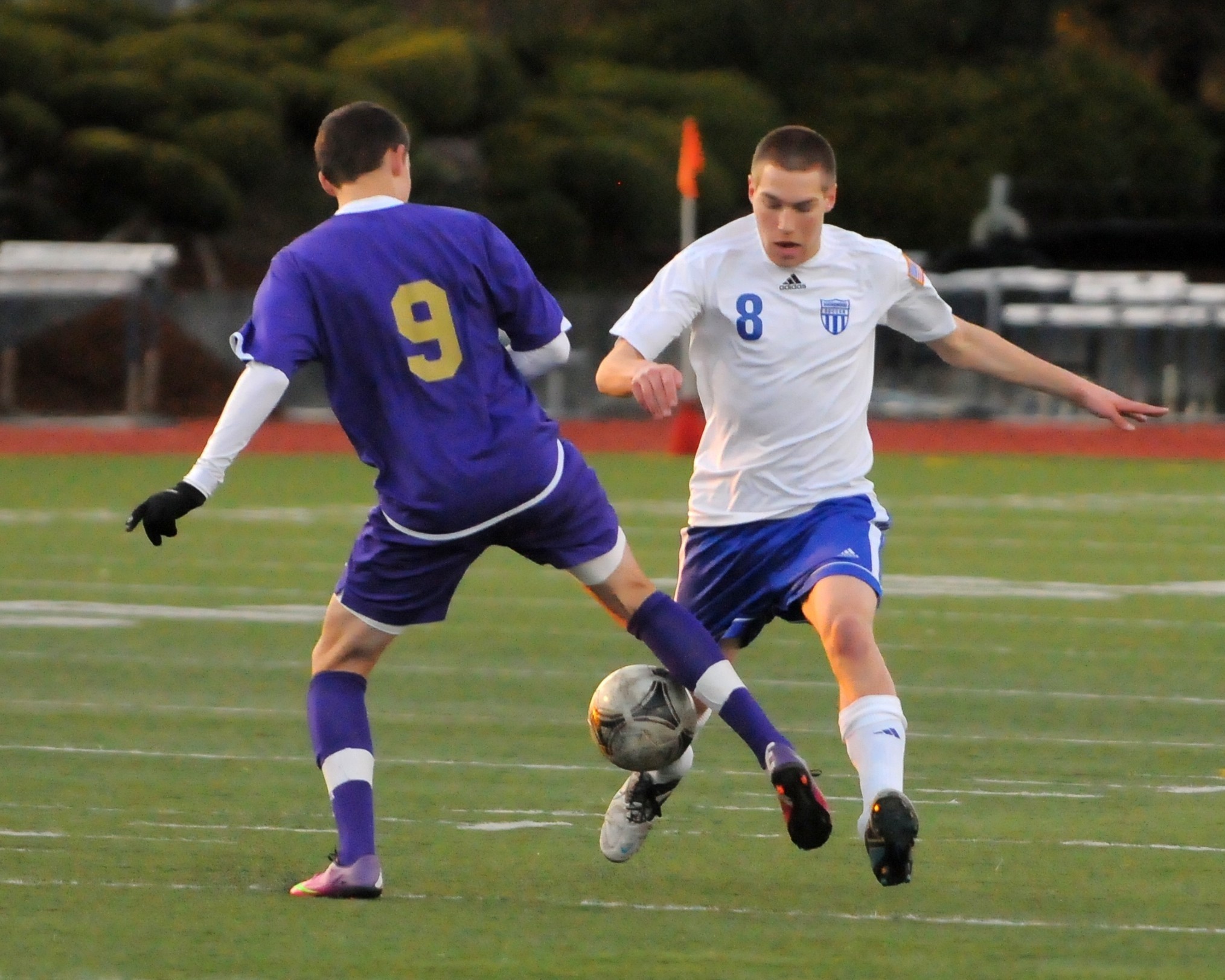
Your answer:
[598, 746, 694, 863]
[866, 791, 920, 887]
[765, 742, 833, 850]
[291, 852, 384, 898]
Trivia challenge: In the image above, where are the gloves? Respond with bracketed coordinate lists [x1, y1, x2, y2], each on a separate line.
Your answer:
[125, 482, 205, 548]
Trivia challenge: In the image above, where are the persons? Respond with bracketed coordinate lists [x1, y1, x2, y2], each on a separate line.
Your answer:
[593, 124, 1171, 886]
[127, 104, 832, 897]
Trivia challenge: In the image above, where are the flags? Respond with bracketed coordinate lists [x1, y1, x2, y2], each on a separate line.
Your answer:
[677, 116, 705, 200]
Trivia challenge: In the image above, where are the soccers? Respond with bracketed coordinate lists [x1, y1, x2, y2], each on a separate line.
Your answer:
[588, 663, 698, 774]
